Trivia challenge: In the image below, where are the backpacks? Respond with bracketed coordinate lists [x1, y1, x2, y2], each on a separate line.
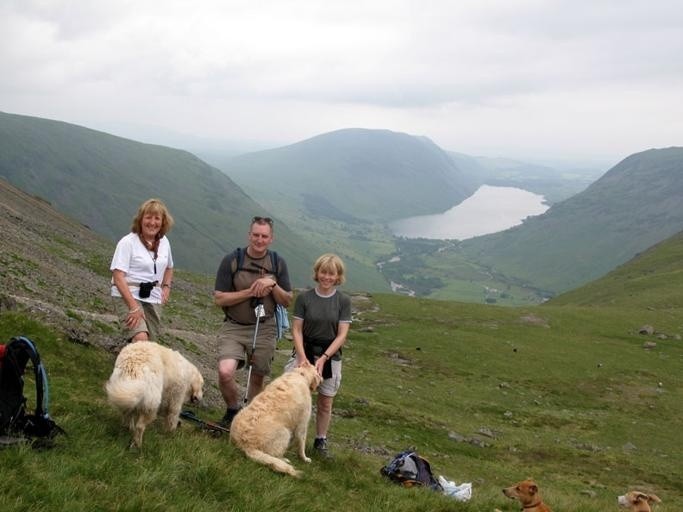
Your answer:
[381, 452, 443, 493]
[0, 334, 56, 442]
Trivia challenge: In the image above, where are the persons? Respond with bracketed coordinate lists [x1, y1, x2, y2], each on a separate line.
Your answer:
[214, 216, 293, 426]
[286, 253, 353, 456]
[110, 198, 175, 342]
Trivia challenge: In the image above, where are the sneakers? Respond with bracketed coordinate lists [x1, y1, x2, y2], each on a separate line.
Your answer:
[313, 437, 333, 460]
[220, 407, 240, 427]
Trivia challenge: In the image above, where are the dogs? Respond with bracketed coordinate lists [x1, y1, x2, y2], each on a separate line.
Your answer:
[102, 339, 205, 450]
[228, 361, 323, 475]
[501, 478, 552, 512]
[617, 490, 662, 511]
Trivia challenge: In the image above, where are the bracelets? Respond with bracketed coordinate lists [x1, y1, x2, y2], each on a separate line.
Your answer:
[323, 352, 330, 360]
[272, 281, 276, 288]
[162, 283, 174, 288]
[128, 305, 141, 314]
